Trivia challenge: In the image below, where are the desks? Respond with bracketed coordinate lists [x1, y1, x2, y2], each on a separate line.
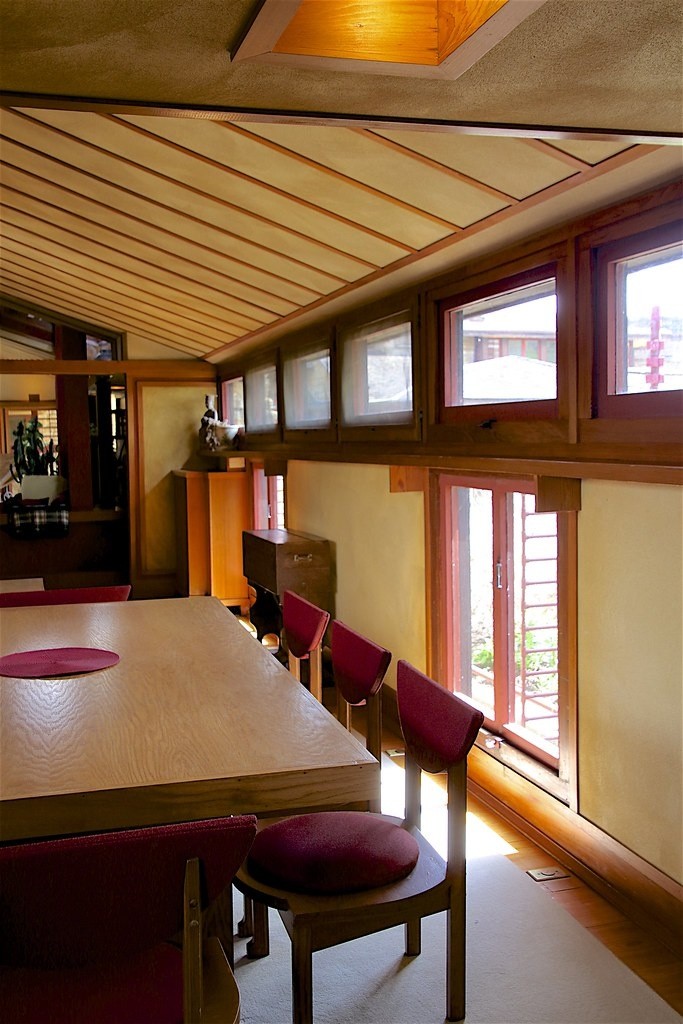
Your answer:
[0, 595, 383, 848]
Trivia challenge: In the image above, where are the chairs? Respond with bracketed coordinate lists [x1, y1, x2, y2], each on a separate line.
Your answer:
[0, 578, 486, 1024]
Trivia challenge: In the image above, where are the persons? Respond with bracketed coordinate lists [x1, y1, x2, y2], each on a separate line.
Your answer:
[199, 394, 218, 445]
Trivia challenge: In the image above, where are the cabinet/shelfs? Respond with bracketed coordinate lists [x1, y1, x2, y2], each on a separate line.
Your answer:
[170, 469, 252, 617]
[243, 527, 333, 630]
